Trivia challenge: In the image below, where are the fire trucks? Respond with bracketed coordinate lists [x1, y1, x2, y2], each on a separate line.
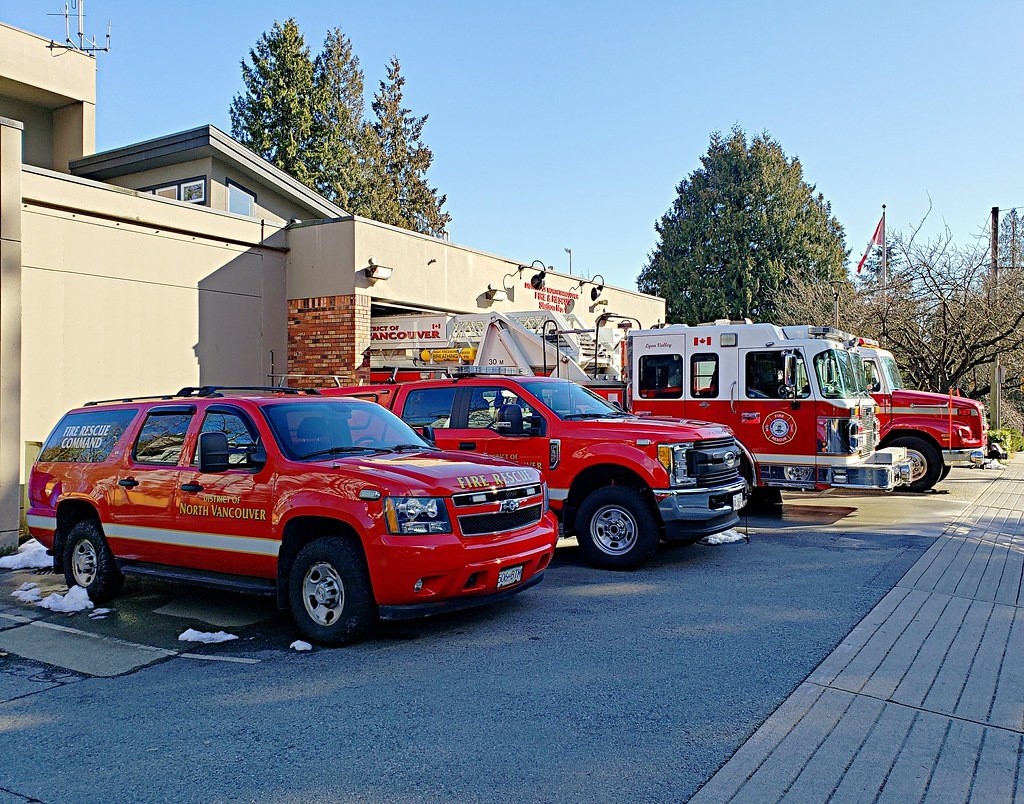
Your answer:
[369, 306, 915, 496]
[695, 335, 991, 493]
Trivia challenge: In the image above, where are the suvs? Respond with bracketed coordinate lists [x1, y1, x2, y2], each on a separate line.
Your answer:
[283, 365, 752, 571]
[23, 384, 561, 646]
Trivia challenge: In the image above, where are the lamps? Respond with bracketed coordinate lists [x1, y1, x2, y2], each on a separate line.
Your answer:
[598, 299, 609, 306]
[518, 260, 547, 291]
[366, 258, 393, 281]
[485, 284, 507, 303]
[579, 275, 605, 301]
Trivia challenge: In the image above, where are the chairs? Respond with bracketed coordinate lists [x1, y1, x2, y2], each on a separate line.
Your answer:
[497, 404, 529, 437]
[295, 417, 330, 455]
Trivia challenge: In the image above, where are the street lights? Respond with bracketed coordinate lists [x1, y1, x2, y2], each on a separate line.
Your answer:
[828, 279, 845, 329]
[564, 248, 572, 275]
